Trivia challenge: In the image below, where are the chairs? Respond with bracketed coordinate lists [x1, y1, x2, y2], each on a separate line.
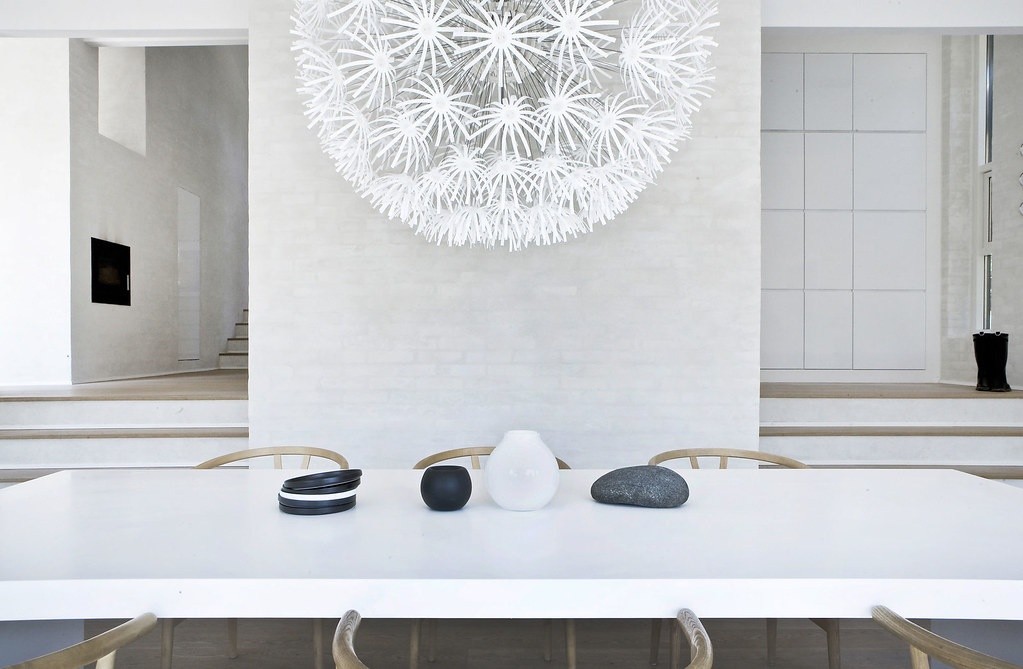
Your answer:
[332, 608, 713, 668]
[407, 446, 578, 669]
[870, 604, 1023, 669]
[155, 443, 349, 669]
[6, 609, 157, 669]
[647, 449, 841, 669]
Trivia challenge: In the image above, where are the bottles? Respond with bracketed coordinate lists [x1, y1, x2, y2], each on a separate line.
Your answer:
[485, 430, 559, 511]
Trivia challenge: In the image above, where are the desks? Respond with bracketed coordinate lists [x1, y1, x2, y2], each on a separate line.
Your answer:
[0, 469, 1022, 620]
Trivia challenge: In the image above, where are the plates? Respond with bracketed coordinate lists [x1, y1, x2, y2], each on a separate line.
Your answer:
[277, 469, 362, 515]
